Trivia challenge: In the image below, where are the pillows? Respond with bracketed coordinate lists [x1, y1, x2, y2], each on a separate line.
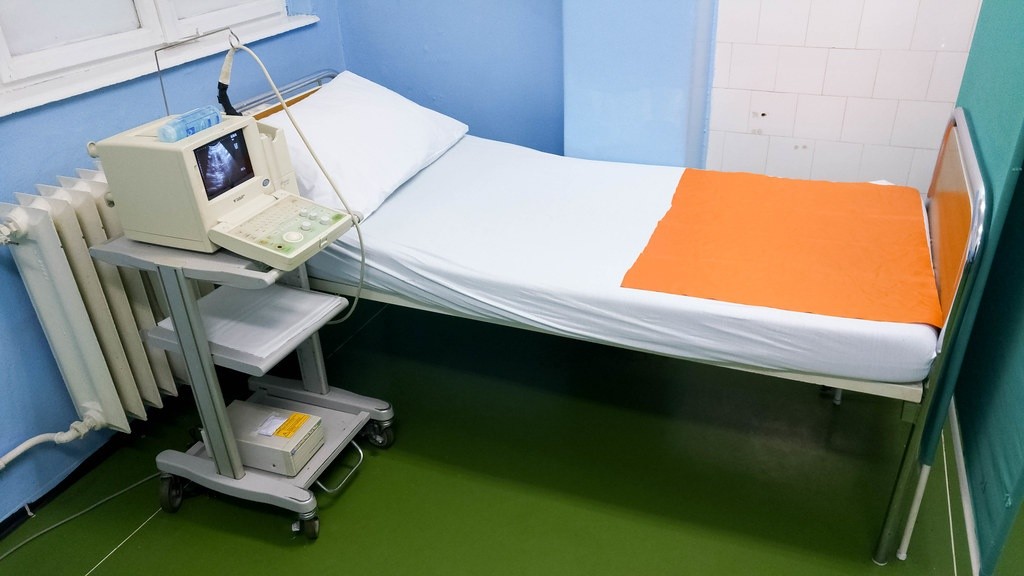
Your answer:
[254, 70, 468, 226]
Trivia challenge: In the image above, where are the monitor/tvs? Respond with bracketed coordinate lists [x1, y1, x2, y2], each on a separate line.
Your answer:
[193, 129, 255, 202]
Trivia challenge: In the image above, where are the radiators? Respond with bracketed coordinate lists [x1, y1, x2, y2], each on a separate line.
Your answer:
[0, 162, 216, 440]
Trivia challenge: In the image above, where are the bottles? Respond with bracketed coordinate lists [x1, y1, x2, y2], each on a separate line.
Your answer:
[158, 105, 222, 142]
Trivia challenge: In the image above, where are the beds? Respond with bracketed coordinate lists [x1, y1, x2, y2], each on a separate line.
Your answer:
[235, 70, 988, 568]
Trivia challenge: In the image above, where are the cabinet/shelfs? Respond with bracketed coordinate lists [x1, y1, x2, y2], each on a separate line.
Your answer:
[90, 232, 396, 540]
